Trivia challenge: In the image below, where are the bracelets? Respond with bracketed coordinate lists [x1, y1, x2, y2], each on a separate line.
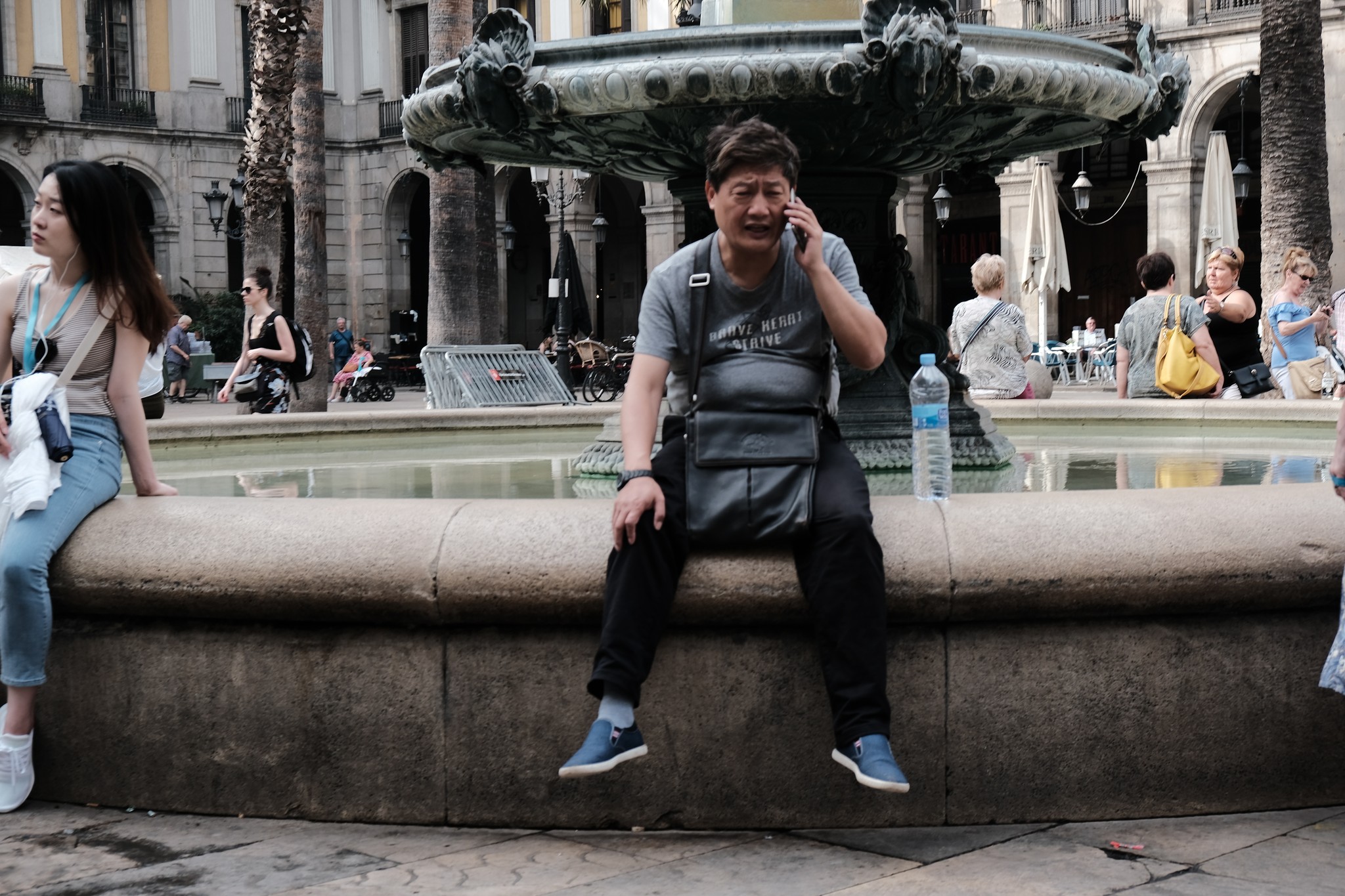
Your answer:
[1330, 475, 1345, 488]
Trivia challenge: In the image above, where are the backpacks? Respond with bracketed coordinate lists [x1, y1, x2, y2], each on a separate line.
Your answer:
[248, 311, 318, 382]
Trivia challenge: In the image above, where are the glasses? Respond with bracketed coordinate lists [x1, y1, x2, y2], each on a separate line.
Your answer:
[238, 287, 265, 294]
[1293, 270, 1314, 282]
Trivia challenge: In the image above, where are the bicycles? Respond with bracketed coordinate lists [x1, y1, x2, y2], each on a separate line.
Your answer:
[582, 333, 637, 403]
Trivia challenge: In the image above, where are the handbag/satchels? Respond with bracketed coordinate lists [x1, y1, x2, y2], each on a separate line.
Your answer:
[343, 362, 358, 373]
[232, 372, 267, 403]
[1232, 362, 1275, 398]
[1155, 294, 1220, 399]
[1327, 343, 1345, 385]
[684, 409, 820, 543]
[1287, 356, 1338, 399]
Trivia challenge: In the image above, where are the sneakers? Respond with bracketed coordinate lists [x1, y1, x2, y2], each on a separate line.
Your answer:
[0, 702, 35, 813]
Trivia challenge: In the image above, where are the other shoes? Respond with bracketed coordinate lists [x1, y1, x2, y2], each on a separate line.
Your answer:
[164, 397, 173, 405]
[177, 398, 192, 404]
[327, 396, 345, 402]
[560, 718, 648, 779]
[831, 735, 910, 794]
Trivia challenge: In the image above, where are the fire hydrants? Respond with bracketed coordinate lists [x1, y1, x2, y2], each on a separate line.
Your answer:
[530, 166, 593, 403]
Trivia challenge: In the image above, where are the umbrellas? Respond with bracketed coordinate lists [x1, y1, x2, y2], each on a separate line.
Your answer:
[543, 228, 592, 401]
[1020, 162, 1071, 366]
[1195, 130, 1239, 296]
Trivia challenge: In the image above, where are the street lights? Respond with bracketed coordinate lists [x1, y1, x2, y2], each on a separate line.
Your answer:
[202, 178, 246, 274]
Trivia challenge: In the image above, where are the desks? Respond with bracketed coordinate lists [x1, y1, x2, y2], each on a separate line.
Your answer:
[1050, 342, 1099, 356]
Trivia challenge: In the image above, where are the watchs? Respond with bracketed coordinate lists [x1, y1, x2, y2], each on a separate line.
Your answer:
[1217, 300, 1225, 315]
[616, 468, 656, 491]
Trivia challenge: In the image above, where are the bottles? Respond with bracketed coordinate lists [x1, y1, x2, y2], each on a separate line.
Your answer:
[1321, 354, 1334, 399]
[908, 354, 953, 502]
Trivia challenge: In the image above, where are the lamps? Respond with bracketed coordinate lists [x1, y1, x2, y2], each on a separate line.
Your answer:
[231, 164, 250, 209]
[203, 174, 228, 244]
[395, 181, 414, 265]
[1072, 148, 1094, 221]
[926, 170, 953, 231]
[498, 165, 515, 254]
[588, 176, 609, 255]
[1227, 71, 1257, 207]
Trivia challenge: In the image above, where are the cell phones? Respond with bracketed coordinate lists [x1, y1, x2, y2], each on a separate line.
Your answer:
[790, 188, 806, 253]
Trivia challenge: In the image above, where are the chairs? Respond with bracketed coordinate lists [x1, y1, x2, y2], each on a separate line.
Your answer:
[1027, 338, 1121, 392]
[573, 336, 618, 366]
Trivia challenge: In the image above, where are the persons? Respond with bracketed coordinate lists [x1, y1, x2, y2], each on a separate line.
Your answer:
[136, 266, 375, 419]
[537, 332, 586, 366]
[1115, 251, 1224, 400]
[1330, 288, 1345, 400]
[1313, 384, 1345, 697]
[1074, 317, 1105, 378]
[1195, 245, 1264, 389]
[0, 157, 182, 814]
[949, 254, 1032, 398]
[1268, 246, 1333, 401]
[558, 115, 910, 795]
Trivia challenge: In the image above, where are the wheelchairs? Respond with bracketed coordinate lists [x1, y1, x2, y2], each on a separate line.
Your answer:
[346, 353, 395, 402]
